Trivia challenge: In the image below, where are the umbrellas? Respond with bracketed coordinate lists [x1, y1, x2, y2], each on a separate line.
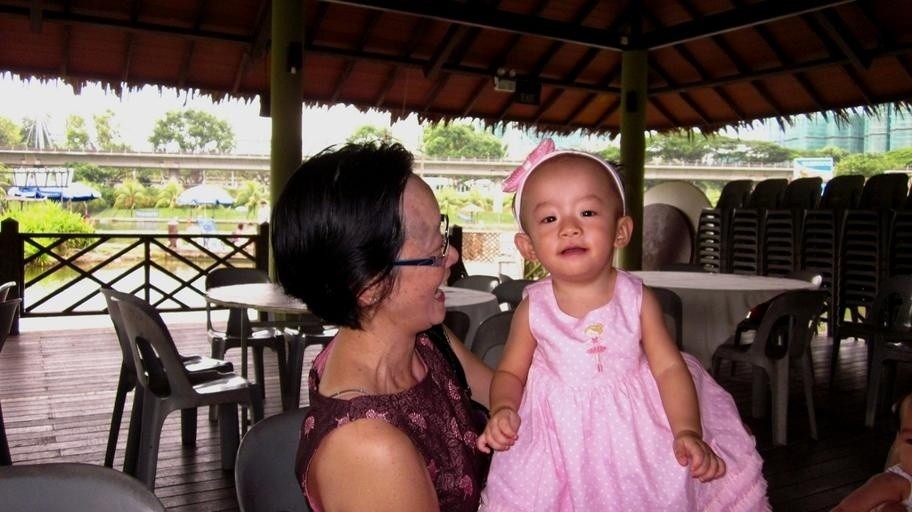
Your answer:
[177, 183, 235, 247]
[61, 182, 104, 201]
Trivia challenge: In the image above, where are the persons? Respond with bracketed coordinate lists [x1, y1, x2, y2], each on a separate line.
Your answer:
[822, 393, 912, 511]
[469, 136, 771, 511]
[269, 146, 502, 512]
[256, 200, 271, 224]
[232, 222, 246, 241]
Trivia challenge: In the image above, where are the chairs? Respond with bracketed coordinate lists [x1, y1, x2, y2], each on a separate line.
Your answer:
[443, 274, 685, 368]
[0, 459, 167, 512]
[831, 277, 911, 427]
[204, 266, 338, 426]
[96, 287, 254, 492]
[235, 408, 320, 512]
[696, 171, 911, 346]
[710, 269, 830, 449]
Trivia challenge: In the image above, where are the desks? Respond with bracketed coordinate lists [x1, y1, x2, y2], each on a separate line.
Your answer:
[617, 269, 816, 375]
[203, 282, 495, 410]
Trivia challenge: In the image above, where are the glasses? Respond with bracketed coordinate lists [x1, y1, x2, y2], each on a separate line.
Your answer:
[389, 213, 451, 276]
